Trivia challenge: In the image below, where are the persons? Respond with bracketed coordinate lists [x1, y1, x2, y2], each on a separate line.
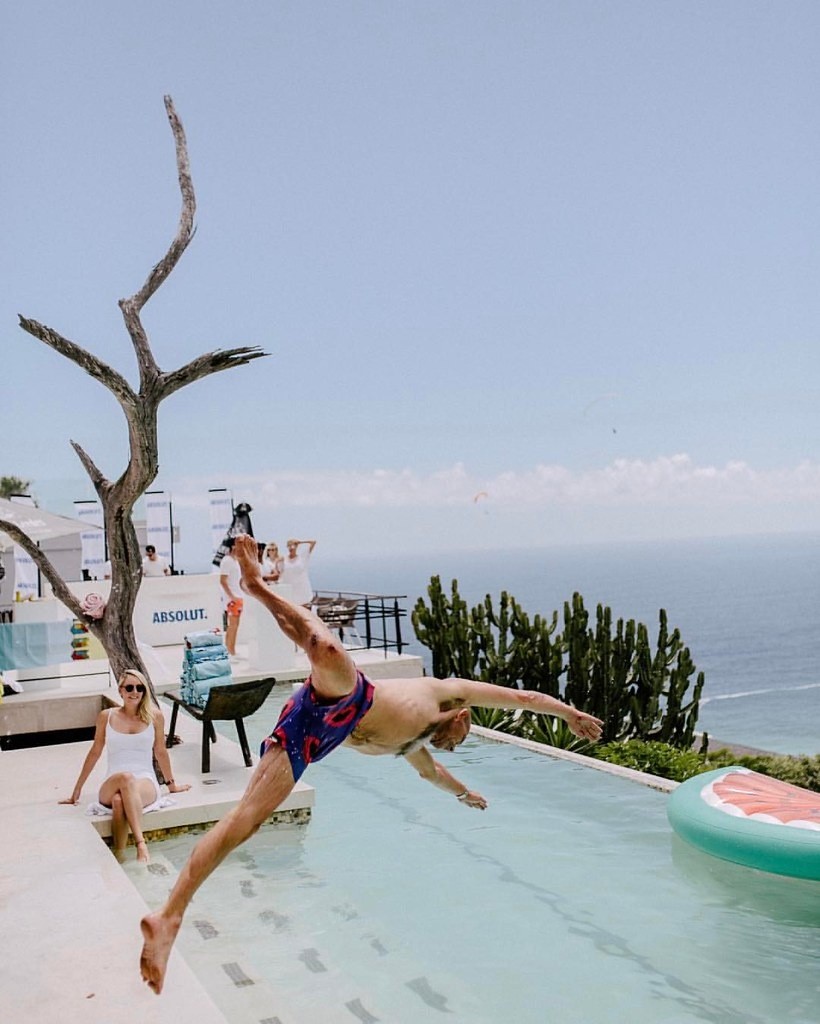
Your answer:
[57, 669, 192, 864]
[220, 537, 242, 611]
[140, 534, 603, 995]
[275, 539, 316, 606]
[257, 543, 279, 584]
[262, 543, 284, 573]
[142, 545, 171, 576]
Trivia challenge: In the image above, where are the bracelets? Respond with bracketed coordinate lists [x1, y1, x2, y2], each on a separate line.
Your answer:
[457, 791, 469, 800]
[166, 780, 174, 785]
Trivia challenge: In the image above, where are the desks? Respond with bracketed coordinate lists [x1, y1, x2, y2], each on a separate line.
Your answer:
[13, 599, 59, 625]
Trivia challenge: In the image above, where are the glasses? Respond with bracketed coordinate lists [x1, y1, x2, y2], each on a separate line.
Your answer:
[120, 684, 145, 692]
[146, 553, 154, 556]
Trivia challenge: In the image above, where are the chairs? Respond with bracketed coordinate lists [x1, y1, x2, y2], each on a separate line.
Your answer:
[163, 677, 278, 774]
[302, 596, 363, 650]
